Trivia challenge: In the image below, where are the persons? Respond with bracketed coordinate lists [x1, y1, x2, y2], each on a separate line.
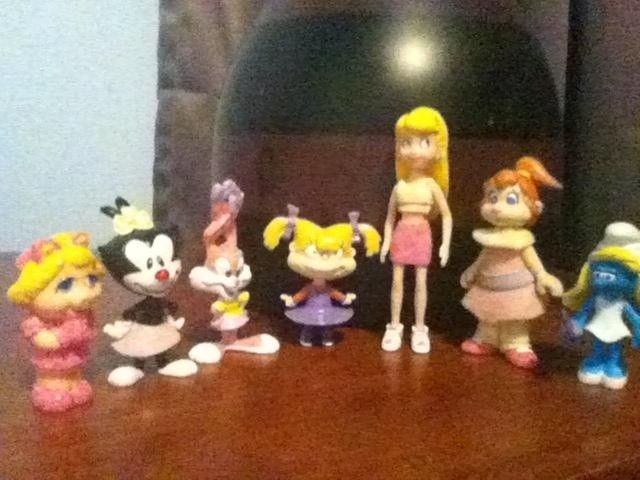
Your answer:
[380, 106, 454, 353]
[457, 157, 563, 369]
[260, 204, 382, 347]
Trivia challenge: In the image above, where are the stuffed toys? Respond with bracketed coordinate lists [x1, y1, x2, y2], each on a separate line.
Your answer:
[188, 177, 280, 363]
[563, 220, 640, 390]
[7, 230, 106, 412]
[95, 197, 198, 387]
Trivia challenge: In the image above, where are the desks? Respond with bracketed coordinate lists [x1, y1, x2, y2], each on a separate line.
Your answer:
[1, 253, 639, 480]
